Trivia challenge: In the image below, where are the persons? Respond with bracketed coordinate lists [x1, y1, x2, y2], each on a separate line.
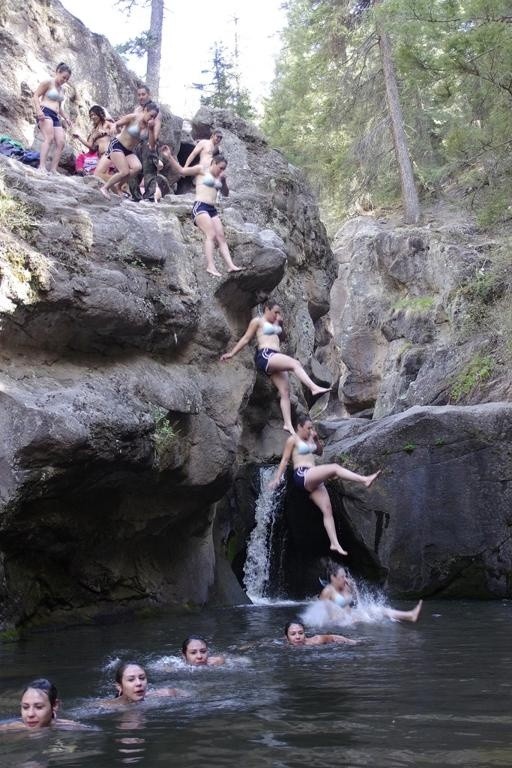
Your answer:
[154, 636, 227, 672]
[92, 649, 177, 710]
[222, 292, 333, 435]
[315, 562, 430, 626]
[33, 60, 73, 175]
[159, 143, 246, 276]
[181, 128, 224, 170]
[71, 85, 179, 204]
[267, 412, 382, 556]
[0, 673, 91, 740]
[237, 617, 360, 651]
